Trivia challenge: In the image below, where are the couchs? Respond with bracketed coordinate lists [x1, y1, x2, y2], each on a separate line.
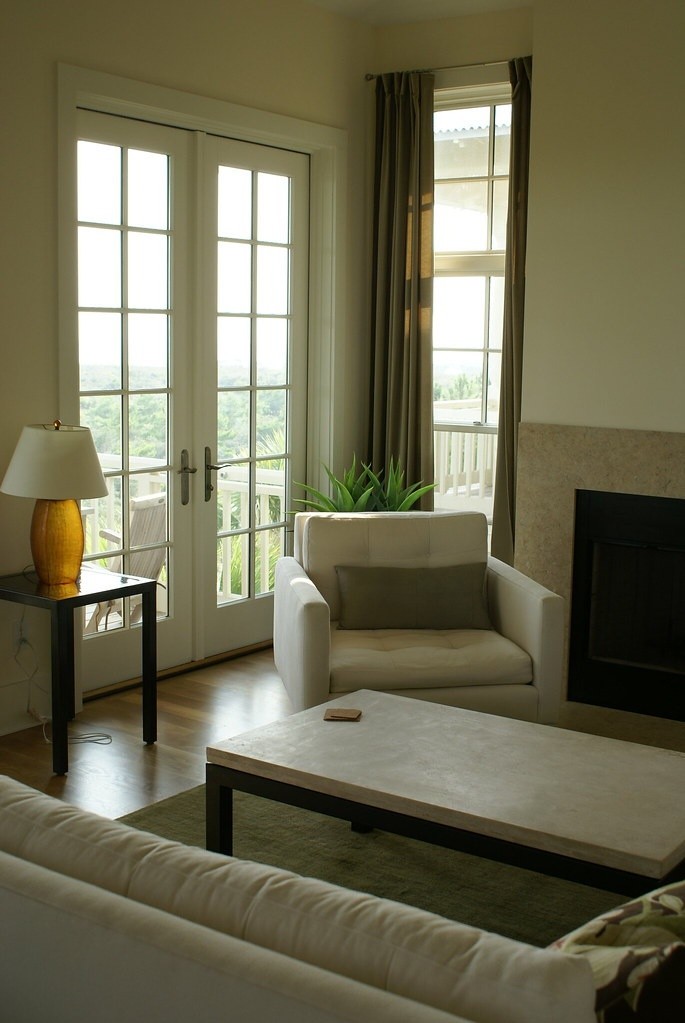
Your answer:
[274, 509, 565, 725]
[1, 777, 603, 1023]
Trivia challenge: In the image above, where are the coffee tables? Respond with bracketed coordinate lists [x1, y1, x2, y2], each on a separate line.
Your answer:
[204, 686, 685, 900]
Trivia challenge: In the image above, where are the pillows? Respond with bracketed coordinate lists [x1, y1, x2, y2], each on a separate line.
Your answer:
[334, 561, 493, 631]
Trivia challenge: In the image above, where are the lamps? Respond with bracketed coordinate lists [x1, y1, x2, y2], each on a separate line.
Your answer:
[0, 419, 109, 585]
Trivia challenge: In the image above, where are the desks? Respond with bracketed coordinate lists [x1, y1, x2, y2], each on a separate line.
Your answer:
[0, 568, 157, 776]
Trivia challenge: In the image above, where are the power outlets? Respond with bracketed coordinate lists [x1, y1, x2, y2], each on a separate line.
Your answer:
[13, 622, 29, 651]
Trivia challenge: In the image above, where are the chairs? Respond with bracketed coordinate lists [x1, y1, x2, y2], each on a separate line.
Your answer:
[81, 492, 168, 635]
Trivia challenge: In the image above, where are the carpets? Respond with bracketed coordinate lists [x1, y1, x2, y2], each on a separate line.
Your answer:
[113, 697, 685, 949]
